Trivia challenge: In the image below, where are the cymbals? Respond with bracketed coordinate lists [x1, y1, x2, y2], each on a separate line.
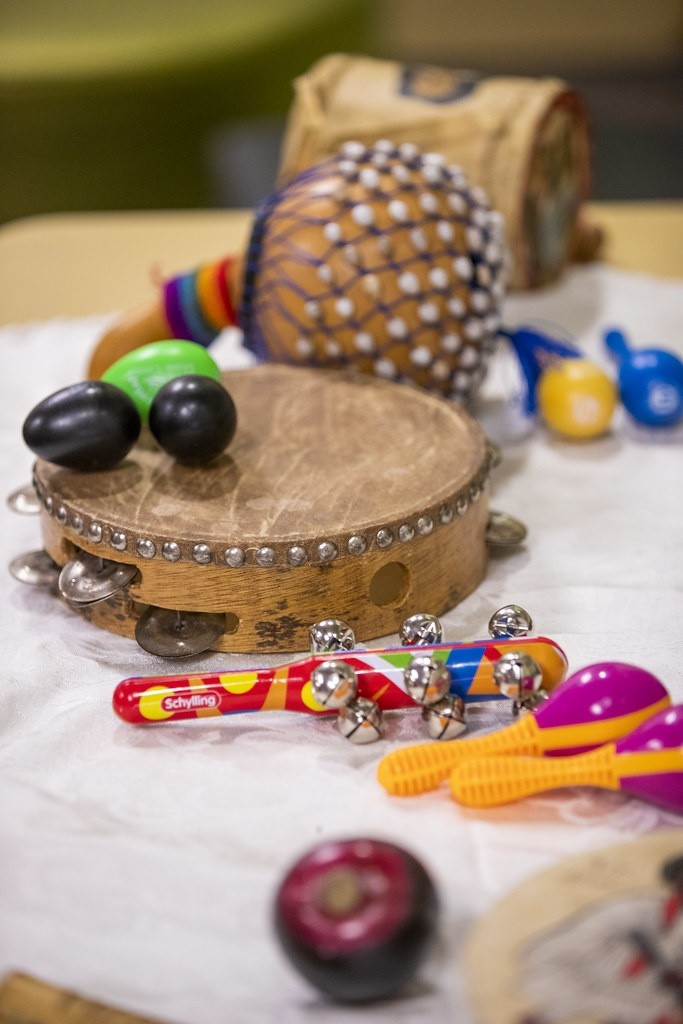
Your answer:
[133, 604, 227, 663]
[6, 483, 41, 518]
[56, 548, 140, 610]
[9, 550, 56, 587]
[482, 507, 530, 550]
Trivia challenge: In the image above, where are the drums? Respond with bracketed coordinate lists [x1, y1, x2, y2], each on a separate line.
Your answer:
[30, 362, 503, 656]
